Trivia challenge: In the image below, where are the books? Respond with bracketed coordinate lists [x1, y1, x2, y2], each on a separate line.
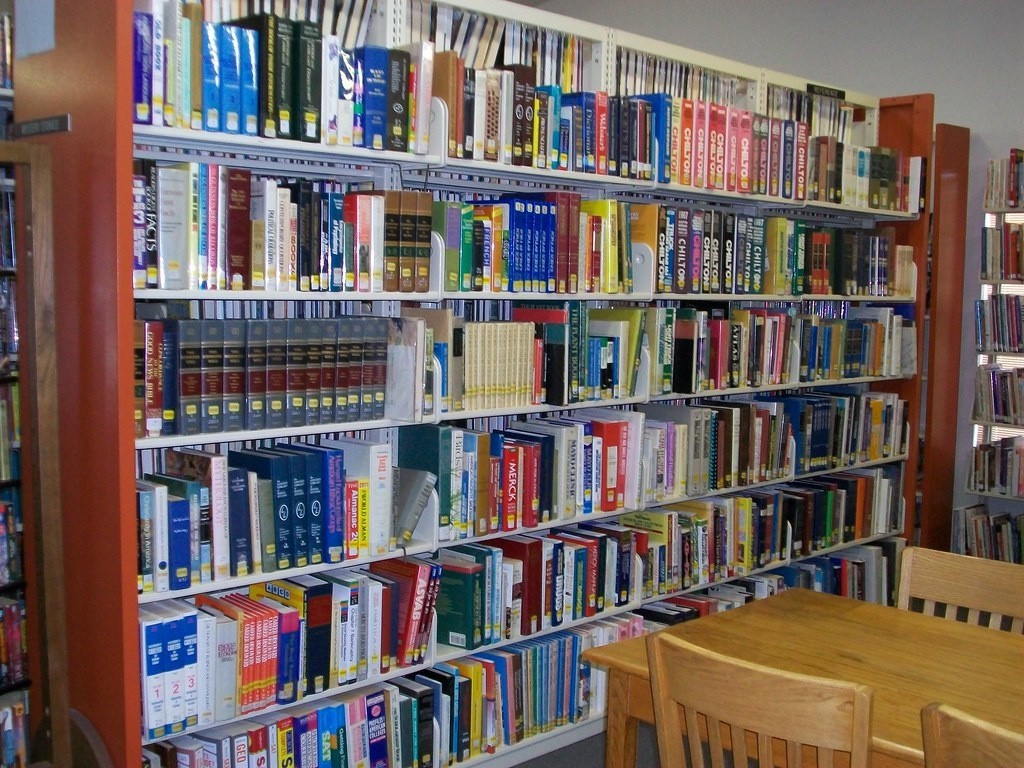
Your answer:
[128, 0, 924, 768]
[953, 147, 1024, 566]
[0, 0, 29, 768]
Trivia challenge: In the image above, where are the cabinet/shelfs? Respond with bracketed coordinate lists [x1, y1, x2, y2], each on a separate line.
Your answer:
[1, 0, 1024, 768]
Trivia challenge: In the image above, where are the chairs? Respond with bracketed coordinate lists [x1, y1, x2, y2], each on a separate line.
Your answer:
[645, 632, 874, 768]
[898, 546, 1024, 634]
[921, 703, 1024, 768]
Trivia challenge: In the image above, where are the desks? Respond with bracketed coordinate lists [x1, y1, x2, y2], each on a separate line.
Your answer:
[581, 586, 1024, 768]
